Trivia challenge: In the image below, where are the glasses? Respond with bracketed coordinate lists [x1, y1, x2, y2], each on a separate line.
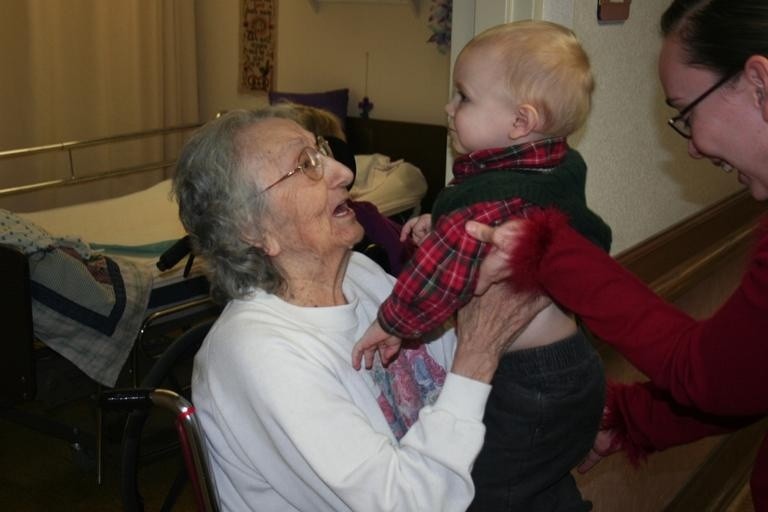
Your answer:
[669, 72, 742, 140]
[253, 133, 334, 192]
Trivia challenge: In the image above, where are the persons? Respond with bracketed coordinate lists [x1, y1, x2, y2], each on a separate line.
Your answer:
[254, 101, 415, 276]
[461, 1, 767, 512]
[350, 16, 611, 512]
[166, 102, 555, 512]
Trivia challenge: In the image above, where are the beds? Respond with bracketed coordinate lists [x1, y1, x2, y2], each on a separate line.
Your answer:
[0, 112, 447, 440]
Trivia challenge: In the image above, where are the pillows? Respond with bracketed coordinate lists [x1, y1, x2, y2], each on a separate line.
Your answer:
[267, 87, 349, 128]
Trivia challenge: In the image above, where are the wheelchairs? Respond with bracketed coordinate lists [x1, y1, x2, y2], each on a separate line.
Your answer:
[93, 231, 232, 511]
